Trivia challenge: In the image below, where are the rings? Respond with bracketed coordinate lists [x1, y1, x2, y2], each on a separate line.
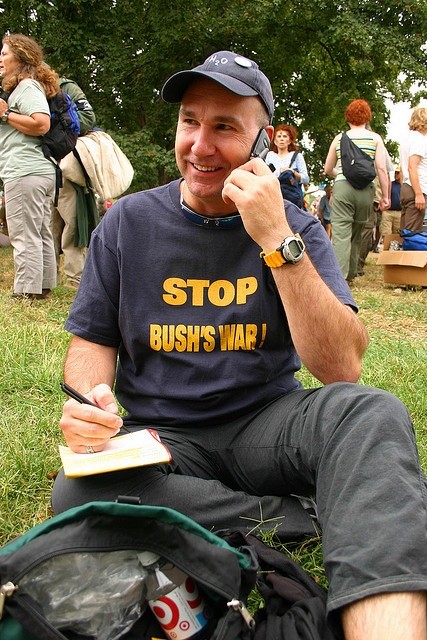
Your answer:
[85, 445, 95, 454]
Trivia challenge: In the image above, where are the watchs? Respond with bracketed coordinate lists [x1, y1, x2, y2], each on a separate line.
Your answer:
[0, 111, 10, 125]
[261, 233, 305, 268]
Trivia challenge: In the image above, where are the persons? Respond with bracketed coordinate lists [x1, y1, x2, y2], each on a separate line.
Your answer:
[49, 50, 426, 639]
[266, 124, 310, 208]
[0, 34, 56, 298]
[325, 99, 389, 282]
[320, 184, 332, 237]
[400, 107, 426, 232]
[358, 147, 391, 276]
[381, 165, 404, 251]
[49, 70, 96, 291]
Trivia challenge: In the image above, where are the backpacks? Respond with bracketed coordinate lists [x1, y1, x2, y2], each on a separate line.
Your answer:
[42, 88, 79, 157]
[0, 495, 330, 640]
[279, 152, 304, 212]
[397, 227, 427, 250]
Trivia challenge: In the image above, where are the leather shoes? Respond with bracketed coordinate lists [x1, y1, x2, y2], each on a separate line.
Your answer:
[12, 293, 46, 300]
[40, 289, 50, 295]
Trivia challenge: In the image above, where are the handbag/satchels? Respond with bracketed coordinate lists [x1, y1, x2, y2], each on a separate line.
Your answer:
[341, 132, 376, 191]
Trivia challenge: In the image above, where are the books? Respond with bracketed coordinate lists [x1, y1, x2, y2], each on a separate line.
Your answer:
[57, 429, 172, 478]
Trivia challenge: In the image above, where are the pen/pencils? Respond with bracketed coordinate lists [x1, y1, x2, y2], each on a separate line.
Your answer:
[60, 382, 131, 433]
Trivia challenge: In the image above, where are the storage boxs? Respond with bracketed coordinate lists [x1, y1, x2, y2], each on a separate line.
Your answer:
[376, 250, 427, 287]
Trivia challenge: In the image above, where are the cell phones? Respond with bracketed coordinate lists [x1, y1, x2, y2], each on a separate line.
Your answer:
[248, 129, 271, 174]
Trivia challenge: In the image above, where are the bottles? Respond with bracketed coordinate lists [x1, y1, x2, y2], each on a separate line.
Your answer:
[135, 551, 216, 639]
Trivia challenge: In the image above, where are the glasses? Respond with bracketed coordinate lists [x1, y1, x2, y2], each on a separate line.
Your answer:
[180, 182, 243, 230]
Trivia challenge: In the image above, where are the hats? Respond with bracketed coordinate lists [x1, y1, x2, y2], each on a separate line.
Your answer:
[161, 50, 274, 123]
